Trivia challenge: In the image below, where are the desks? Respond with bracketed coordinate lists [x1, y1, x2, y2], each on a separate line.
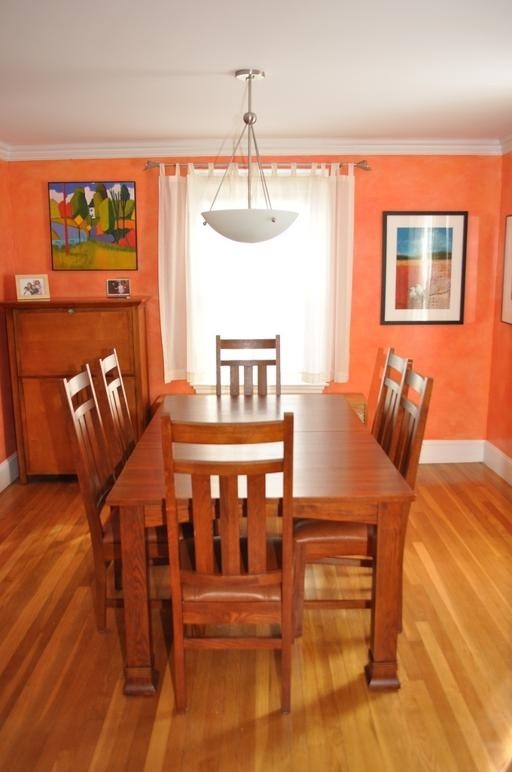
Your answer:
[104, 392, 416, 698]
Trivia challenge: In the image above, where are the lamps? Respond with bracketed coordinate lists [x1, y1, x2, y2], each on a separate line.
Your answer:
[201, 69, 298, 243]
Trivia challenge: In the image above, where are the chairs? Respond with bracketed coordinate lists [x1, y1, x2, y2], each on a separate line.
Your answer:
[59, 363, 207, 639]
[215, 335, 281, 397]
[295, 366, 433, 636]
[95, 349, 139, 478]
[160, 412, 297, 715]
[368, 349, 413, 456]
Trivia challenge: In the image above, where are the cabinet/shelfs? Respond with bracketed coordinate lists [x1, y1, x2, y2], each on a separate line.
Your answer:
[0, 300, 150, 484]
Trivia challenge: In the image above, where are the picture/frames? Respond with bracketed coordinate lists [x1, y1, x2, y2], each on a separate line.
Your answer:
[380, 211, 469, 325]
[15, 274, 50, 301]
[501, 215, 512, 325]
[106, 279, 132, 298]
[48, 181, 138, 271]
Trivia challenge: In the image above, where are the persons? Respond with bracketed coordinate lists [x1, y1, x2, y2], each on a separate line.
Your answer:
[23, 282, 32, 295]
[33, 280, 41, 294]
[114, 282, 124, 293]
[109, 282, 114, 293]
